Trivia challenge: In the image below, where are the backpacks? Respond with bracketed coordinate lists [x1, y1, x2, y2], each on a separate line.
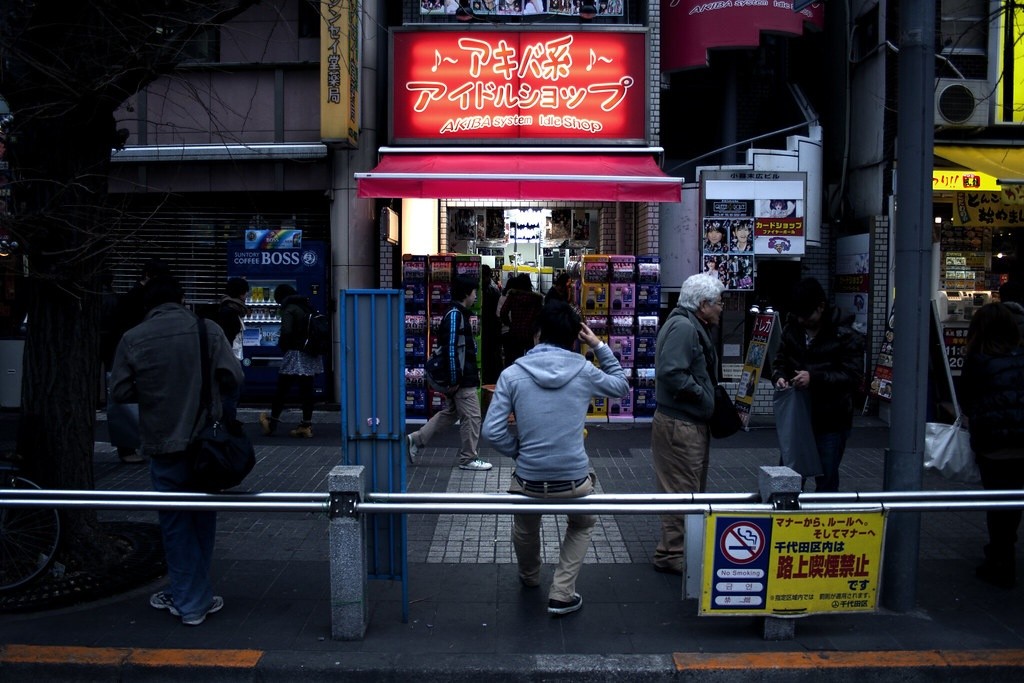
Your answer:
[295, 307, 330, 357]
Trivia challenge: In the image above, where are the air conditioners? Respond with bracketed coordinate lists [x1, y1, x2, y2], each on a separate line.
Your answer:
[934, 79, 990, 124]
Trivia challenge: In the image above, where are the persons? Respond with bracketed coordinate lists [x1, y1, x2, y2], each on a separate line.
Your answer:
[771, 278, 864, 492]
[483, 264, 573, 384]
[652, 274, 726, 572]
[218, 276, 250, 407]
[102, 270, 152, 464]
[706, 219, 753, 289]
[958, 281, 1024, 590]
[260, 285, 324, 437]
[109, 270, 244, 623]
[481, 301, 630, 613]
[746, 345, 759, 397]
[405, 273, 493, 470]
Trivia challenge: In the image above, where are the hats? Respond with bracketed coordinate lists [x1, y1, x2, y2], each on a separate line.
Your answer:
[785, 279, 824, 318]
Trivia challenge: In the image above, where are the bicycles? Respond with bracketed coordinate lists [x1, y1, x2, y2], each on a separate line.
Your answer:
[0, 461, 60, 590]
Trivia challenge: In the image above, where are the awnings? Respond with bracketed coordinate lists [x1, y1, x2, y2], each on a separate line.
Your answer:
[933, 146, 1024, 184]
[354, 155, 682, 203]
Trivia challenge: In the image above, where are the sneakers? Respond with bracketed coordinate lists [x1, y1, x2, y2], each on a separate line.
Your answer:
[148, 591, 225, 626]
[290, 424, 315, 439]
[458, 458, 494, 471]
[260, 411, 274, 435]
[548, 593, 585, 615]
[406, 432, 420, 463]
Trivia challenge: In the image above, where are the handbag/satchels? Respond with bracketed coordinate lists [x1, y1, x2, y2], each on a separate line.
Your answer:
[709, 385, 743, 439]
[771, 385, 824, 480]
[426, 355, 456, 393]
[183, 418, 257, 492]
[924, 423, 981, 485]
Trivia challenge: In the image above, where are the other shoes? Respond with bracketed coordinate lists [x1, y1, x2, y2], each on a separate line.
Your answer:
[652, 550, 685, 574]
[976, 559, 1019, 588]
[120, 452, 146, 463]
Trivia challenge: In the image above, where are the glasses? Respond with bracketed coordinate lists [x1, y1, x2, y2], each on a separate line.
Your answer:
[707, 299, 727, 309]
